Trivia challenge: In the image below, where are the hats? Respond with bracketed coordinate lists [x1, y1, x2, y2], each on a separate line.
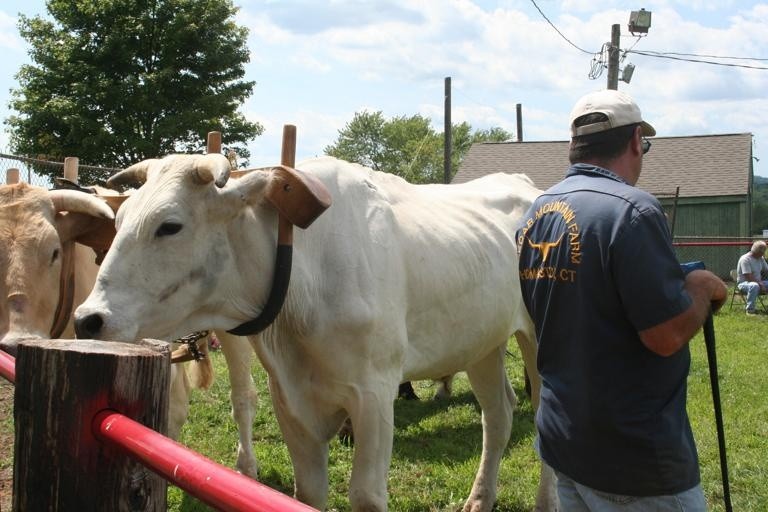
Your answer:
[569, 90, 656, 138]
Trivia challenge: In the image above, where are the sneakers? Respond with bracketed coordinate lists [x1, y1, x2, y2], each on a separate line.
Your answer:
[745, 308, 762, 315]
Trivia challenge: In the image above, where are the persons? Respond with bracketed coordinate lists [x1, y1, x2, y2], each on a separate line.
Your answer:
[516, 90, 732, 511]
[736, 240, 768, 316]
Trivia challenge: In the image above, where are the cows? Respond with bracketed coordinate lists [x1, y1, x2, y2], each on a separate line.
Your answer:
[2, 178, 260, 479]
[74, 152, 549, 512]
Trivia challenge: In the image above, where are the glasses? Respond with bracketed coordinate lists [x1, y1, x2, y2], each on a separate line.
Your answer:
[640, 137, 651, 155]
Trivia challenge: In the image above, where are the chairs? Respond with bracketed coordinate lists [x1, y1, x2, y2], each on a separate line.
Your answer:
[730, 269, 768, 313]
